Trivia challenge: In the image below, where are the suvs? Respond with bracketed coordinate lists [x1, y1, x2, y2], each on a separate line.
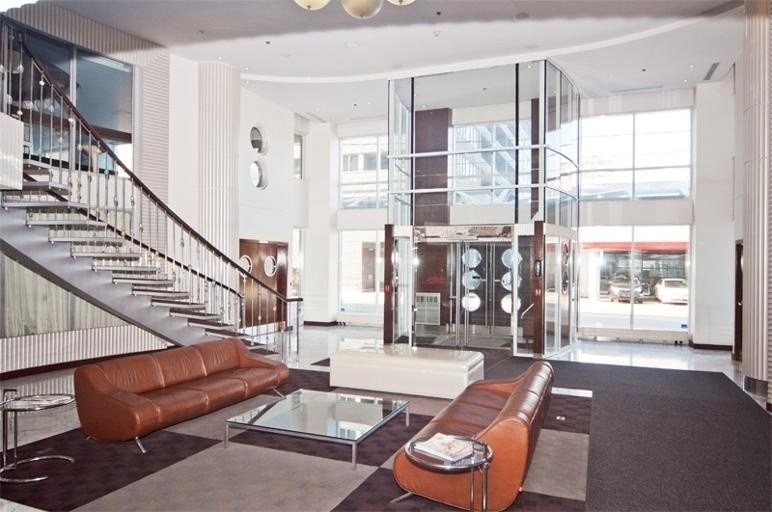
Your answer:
[608, 273, 644, 302]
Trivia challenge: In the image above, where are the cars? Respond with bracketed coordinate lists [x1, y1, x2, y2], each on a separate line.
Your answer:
[654, 277, 689, 304]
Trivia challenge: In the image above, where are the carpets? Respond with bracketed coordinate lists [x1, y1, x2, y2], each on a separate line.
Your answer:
[1, 341, 772, 512]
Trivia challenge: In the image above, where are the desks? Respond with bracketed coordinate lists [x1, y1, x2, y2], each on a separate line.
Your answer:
[406, 435, 495, 512]
[0, 388, 76, 486]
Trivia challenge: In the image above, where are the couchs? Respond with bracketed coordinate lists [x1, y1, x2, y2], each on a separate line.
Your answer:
[391, 358, 558, 512]
[72, 336, 291, 460]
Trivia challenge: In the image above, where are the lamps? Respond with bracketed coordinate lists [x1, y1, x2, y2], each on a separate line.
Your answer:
[294, 1, 417, 22]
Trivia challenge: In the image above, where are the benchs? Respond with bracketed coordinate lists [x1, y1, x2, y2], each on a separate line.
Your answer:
[328, 344, 486, 402]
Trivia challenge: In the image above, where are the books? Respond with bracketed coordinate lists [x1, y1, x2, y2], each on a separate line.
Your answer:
[414, 432, 474, 462]
